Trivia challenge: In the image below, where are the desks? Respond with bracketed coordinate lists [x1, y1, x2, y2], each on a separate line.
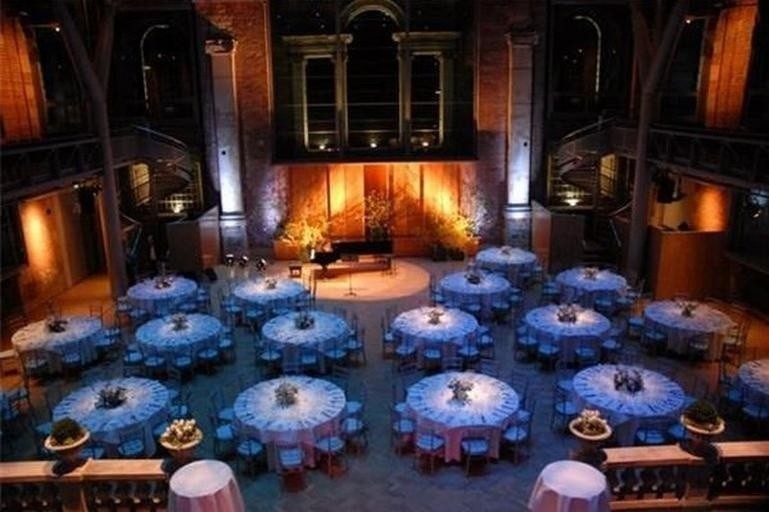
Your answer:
[165, 459, 242, 512]
[529, 459, 610, 511]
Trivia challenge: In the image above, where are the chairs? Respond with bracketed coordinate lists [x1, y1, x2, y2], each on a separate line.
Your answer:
[380, 244, 768, 480]
[0, 266, 369, 494]
